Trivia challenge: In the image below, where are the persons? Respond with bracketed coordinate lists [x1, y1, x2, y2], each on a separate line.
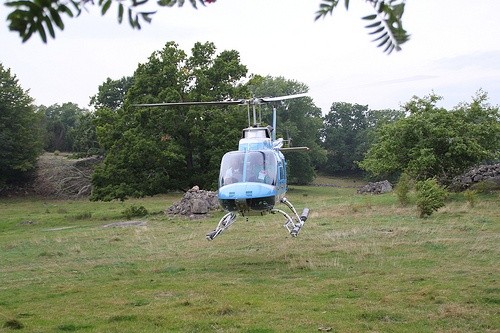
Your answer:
[254, 157, 275, 181]
[223, 160, 243, 184]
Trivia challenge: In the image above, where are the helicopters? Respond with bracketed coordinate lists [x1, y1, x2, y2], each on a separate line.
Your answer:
[130, 93, 310, 243]
